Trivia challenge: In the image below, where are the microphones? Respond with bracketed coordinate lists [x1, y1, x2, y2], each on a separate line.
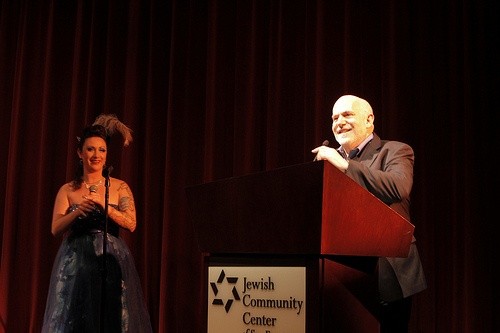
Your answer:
[313, 140, 329, 161]
[90, 185, 98, 195]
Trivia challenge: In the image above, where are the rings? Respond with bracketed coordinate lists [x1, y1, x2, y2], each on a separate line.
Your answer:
[85, 196, 87, 199]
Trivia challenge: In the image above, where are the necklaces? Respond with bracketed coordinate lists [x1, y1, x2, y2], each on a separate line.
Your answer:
[80, 175, 106, 188]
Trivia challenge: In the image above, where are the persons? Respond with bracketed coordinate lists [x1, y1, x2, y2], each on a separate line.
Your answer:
[44, 125, 137, 333]
[313, 94, 426, 333]
